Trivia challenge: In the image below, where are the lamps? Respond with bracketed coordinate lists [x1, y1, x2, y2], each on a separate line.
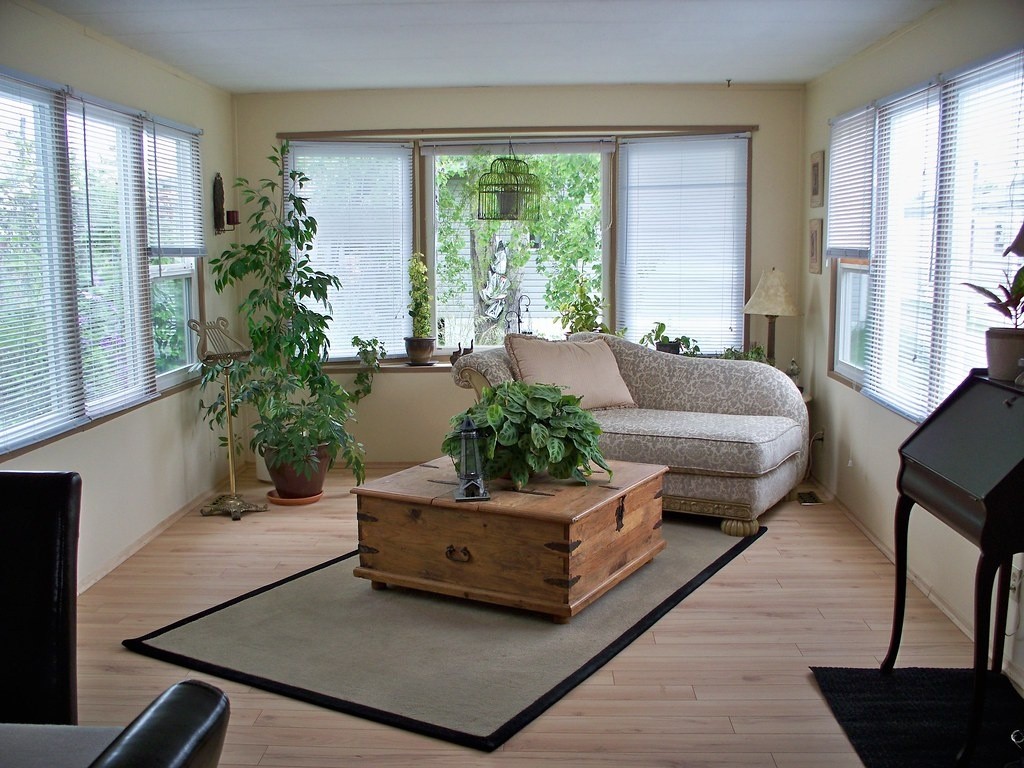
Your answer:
[742, 271, 804, 365]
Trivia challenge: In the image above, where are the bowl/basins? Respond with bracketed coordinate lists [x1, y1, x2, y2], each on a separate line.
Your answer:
[498, 194, 523, 214]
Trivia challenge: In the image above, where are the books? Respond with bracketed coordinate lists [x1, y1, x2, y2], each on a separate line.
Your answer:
[797, 490, 825, 506]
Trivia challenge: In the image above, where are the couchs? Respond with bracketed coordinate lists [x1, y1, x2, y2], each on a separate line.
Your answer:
[451, 331, 809, 520]
[0, 681, 232, 768]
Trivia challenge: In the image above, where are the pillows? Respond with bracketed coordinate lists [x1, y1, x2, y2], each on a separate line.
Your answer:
[502, 334, 640, 416]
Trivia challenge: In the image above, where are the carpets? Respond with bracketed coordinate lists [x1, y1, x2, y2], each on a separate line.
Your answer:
[809, 664, 1024, 768]
[123, 493, 769, 751]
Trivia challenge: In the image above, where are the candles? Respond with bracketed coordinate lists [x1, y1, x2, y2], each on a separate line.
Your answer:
[227, 211, 239, 224]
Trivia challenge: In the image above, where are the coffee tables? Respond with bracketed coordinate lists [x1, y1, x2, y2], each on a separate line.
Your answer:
[349, 446, 668, 625]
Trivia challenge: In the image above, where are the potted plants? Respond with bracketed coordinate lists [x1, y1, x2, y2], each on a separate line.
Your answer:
[553, 276, 611, 340]
[188, 138, 388, 505]
[639, 322, 700, 357]
[403, 252, 439, 365]
[960, 271, 1023, 380]
[442, 381, 615, 489]
